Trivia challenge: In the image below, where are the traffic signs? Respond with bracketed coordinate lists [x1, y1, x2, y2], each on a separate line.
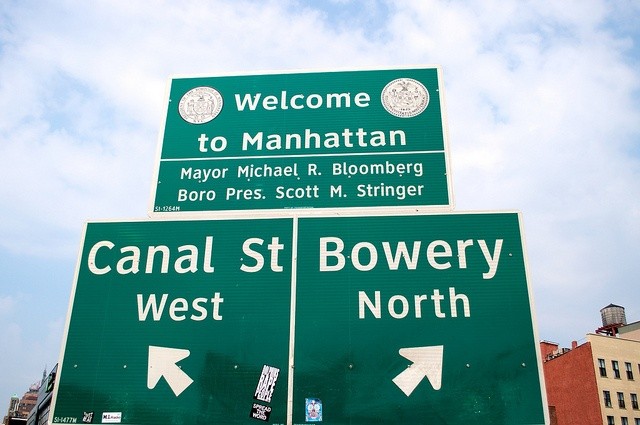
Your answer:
[152, 68, 452, 213]
[47, 211, 551, 425]
